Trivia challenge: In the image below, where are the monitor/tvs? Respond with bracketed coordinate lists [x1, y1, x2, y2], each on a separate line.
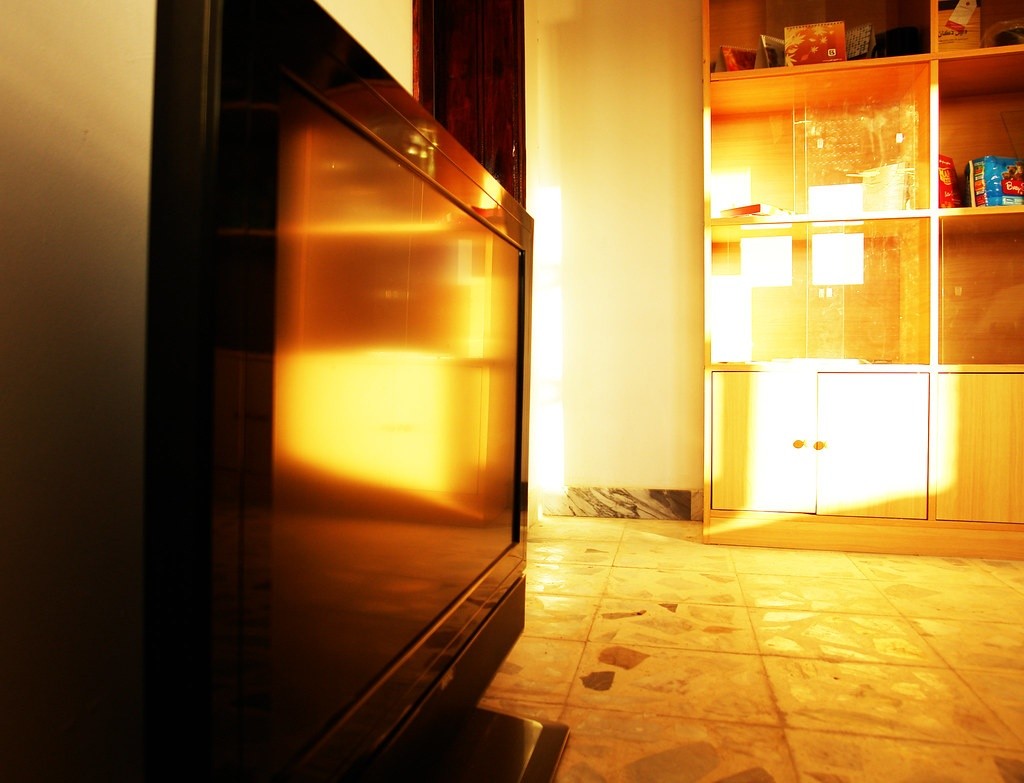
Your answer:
[138, 0, 543, 783]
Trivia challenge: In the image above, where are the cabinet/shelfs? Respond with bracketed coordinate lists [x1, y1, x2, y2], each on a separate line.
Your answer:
[700, 0, 1024, 561]
